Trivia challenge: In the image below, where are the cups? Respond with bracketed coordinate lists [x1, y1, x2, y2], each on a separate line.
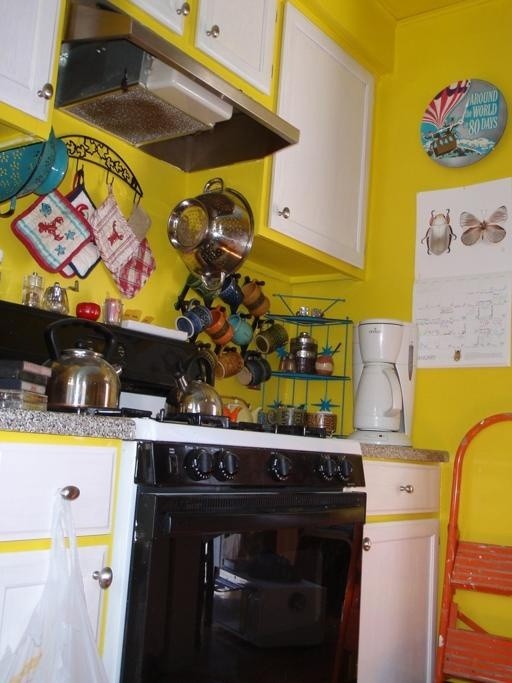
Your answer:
[266, 408, 308, 425]
[241, 278, 270, 315]
[104, 298, 123, 326]
[304, 413, 339, 433]
[213, 347, 244, 378]
[236, 351, 271, 386]
[187, 272, 215, 298]
[175, 298, 213, 338]
[256, 320, 289, 354]
[218, 276, 243, 306]
[204, 304, 234, 346]
[228, 314, 253, 346]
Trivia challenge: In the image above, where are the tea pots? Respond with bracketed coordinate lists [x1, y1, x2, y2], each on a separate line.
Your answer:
[164, 373, 225, 415]
[41, 318, 123, 415]
[223, 400, 263, 424]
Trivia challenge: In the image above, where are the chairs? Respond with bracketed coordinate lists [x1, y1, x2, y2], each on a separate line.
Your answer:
[434, 414, 512, 682]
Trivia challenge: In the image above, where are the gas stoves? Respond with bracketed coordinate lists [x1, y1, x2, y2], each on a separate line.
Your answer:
[120, 406, 365, 486]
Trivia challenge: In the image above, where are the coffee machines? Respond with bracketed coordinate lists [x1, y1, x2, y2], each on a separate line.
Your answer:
[352, 321, 416, 447]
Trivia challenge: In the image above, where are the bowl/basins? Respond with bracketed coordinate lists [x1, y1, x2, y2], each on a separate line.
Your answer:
[76, 302, 100, 322]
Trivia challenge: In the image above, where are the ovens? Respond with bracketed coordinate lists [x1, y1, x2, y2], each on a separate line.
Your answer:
[120, 490, 368, 681]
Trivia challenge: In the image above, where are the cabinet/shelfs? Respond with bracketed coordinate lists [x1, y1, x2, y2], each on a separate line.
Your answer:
[0, 0, 69, 155]
[258, 299, 351, 436]
[360, 443, 447, 683]
[3, 414, 127, 683]
[186, 2, 377, 281]
[116, 1, 276, 110]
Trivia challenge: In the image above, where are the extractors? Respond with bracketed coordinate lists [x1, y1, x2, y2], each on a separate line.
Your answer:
[53, 0, 300, 174]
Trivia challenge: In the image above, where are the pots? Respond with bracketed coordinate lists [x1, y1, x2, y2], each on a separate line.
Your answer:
[0, 125, 69, 218]
[167, 177, 255, 290]
